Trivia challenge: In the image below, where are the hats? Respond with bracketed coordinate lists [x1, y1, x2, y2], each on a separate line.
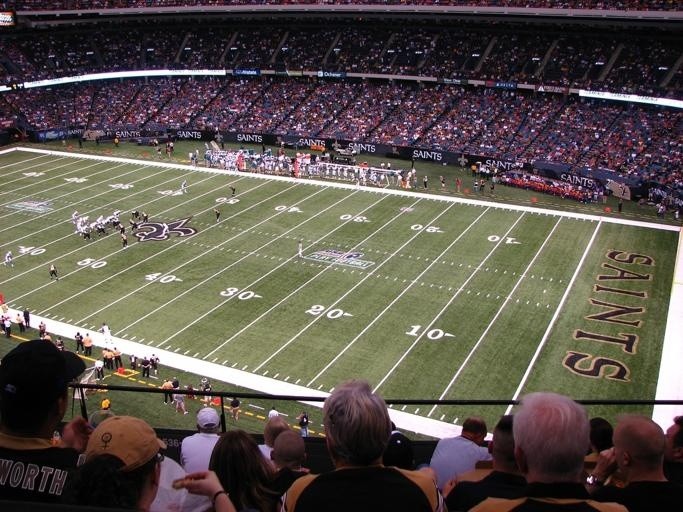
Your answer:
[198, 407, 220, 429]
[0, 339, 85, 394]
[87, 415, 167, 471]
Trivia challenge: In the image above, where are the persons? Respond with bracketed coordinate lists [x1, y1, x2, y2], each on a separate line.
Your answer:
[48, 263, 58, 281]
[0, 0, 683, 248]
[0, 307, 683, 512]
